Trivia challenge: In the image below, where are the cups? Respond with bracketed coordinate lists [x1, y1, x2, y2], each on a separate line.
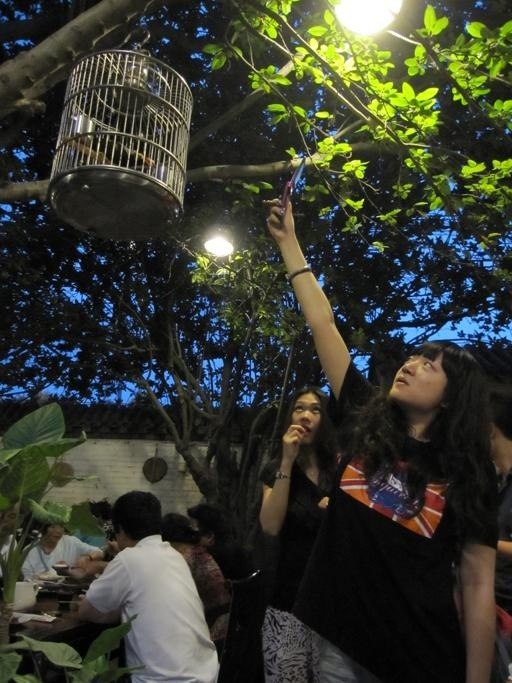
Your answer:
[12, 580, 40, 612]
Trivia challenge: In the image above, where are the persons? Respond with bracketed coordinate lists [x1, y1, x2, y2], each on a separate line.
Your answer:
[0, 383, 511, 682]
[0, 505, 105, 589]
[451, 385, 510, 683]
[260, 387, 355, 681]
[264, 189, 501, 681]
[78, 491, 238, 681]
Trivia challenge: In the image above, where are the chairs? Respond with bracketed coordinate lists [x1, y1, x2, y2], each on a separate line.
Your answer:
[218, 568, 263, 683]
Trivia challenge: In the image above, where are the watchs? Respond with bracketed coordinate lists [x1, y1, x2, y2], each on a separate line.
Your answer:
[275, 472, 291, 480]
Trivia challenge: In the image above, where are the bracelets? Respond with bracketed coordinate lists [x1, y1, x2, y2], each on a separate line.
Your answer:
[288, 264, 312, 286]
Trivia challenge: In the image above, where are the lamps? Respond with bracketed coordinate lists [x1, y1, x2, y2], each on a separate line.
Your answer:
[201, 181, 237, 258]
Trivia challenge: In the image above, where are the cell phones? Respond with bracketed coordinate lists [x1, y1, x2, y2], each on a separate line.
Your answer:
[280, 155, 306, 215]
